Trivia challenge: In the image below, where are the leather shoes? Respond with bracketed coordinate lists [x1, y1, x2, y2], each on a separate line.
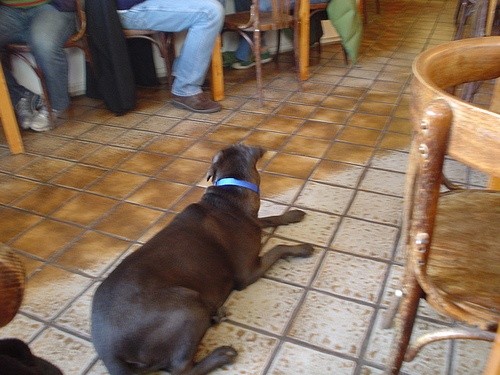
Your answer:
[200, 79, 211, 89]
[171, 93, 223, 113]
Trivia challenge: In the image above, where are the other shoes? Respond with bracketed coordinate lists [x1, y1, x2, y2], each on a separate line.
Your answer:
[232, 50, 272, 69]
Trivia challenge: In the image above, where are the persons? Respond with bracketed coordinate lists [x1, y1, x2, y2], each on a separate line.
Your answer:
[0, 0, 81, 131]
[232, 0, 329, 69]
[115, 0, 225, 114]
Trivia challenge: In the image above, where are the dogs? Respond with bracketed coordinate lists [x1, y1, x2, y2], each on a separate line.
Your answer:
[90, 144, 315, 375]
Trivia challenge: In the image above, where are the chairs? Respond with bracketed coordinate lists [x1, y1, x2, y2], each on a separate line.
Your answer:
[0, 0, 379, 131]
[386, 35, 500, 375]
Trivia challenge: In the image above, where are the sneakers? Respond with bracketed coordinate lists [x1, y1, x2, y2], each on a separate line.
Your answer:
[30, 103, 65, 132]
[15, 92, 37, 129]
